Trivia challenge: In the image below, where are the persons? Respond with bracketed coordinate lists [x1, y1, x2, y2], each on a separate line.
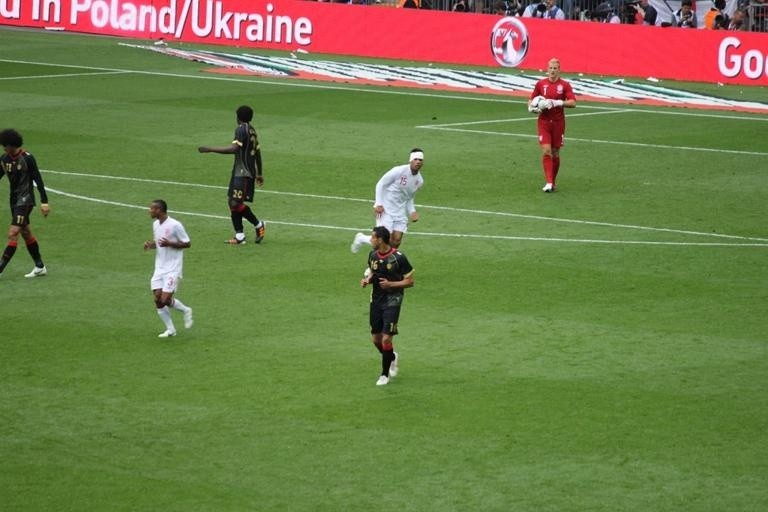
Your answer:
[350, 148, 424, 278]
[528, 56, 578, 192]
[143, 198, 194, 337]
[312, 1, 768, 31]
[0, 127, 52, 281]
[198, 104, 266, 245]
[360, 225, 417, 387]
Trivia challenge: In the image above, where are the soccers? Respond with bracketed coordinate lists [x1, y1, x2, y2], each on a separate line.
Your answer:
[531, 93, 546, 112]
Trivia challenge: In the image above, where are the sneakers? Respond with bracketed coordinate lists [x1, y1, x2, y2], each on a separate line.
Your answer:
[376, 375, 390, 385]
[183, 307, 194, 328]
[351, 232, 364, 253]
[224, 237, 247, 245]
[157, 329, 177, 338]
[24, 266, 47, 278]
[255, 220, 266, 243]
[364, 267, 371, 278]
[389, 352, 398, 377]
[542, 183, 552, 192]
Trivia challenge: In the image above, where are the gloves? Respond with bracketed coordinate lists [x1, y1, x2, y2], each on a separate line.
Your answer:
[543, 98, 563, 110]
[528, 106, 542, 113]
